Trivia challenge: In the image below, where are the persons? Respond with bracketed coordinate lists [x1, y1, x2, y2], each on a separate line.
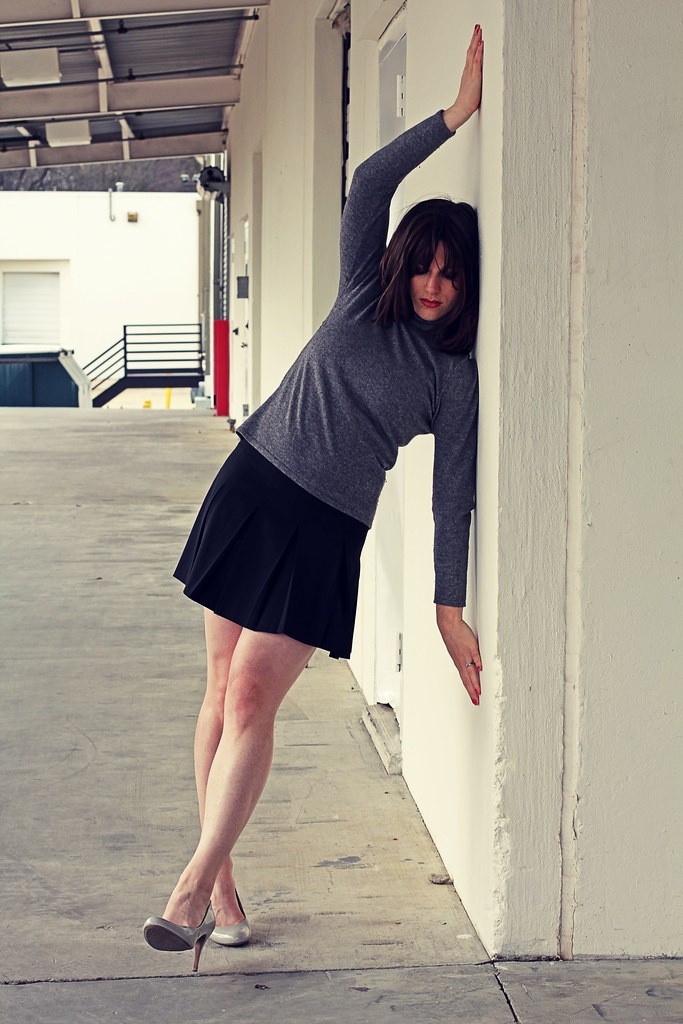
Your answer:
[141, 24, 483, 973]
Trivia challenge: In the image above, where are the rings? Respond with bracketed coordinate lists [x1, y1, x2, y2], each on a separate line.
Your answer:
[466, 662, 474, 669]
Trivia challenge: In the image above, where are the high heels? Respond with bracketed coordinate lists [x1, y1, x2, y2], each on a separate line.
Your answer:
[142, 900, 216, 972]
[209, 887, 252, 945]
